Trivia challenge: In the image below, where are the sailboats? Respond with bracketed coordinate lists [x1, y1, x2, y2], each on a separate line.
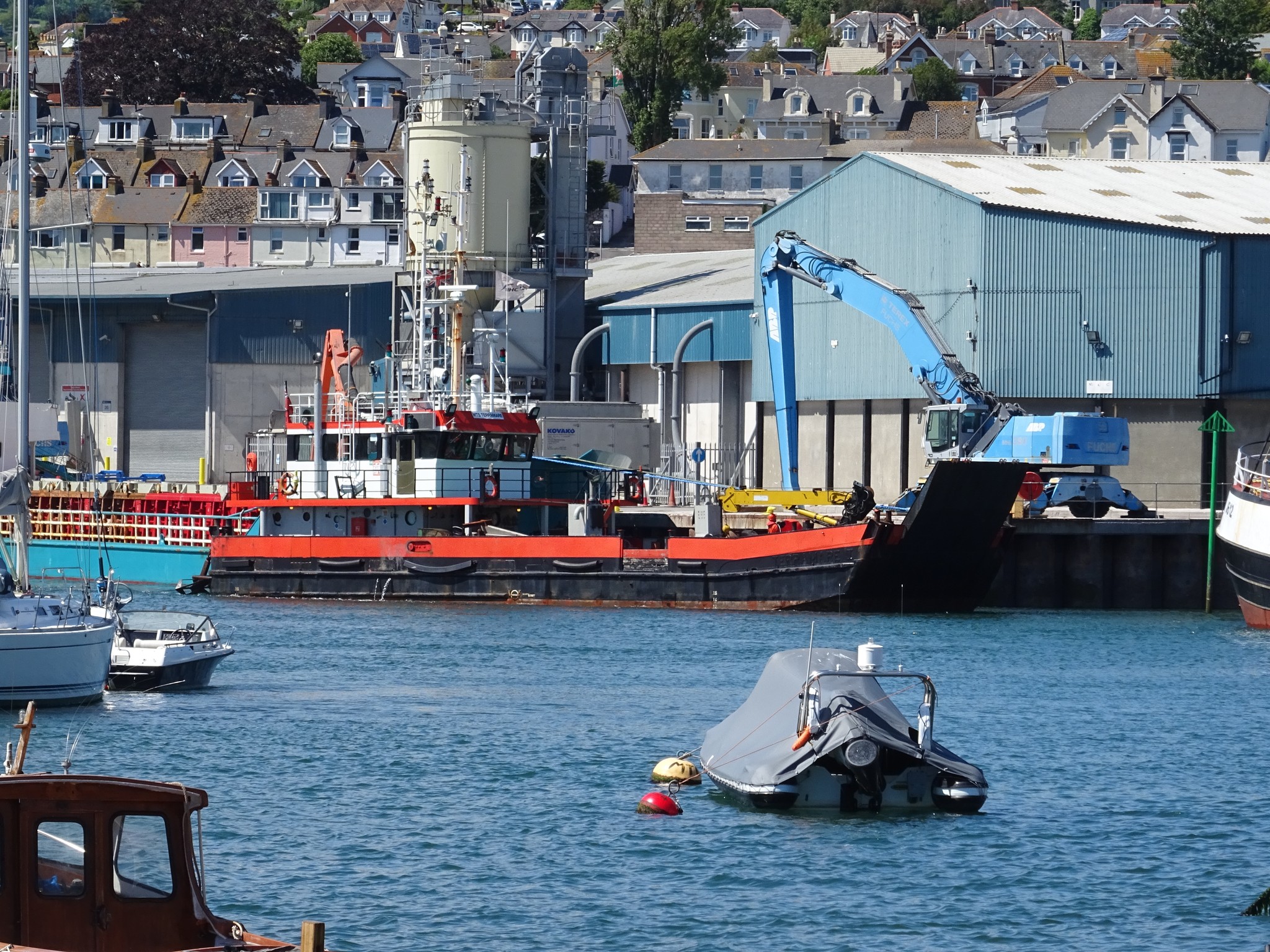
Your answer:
[0, 0, 129, 707]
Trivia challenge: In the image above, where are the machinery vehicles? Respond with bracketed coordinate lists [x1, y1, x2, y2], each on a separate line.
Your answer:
[758, 228, 1167, 527]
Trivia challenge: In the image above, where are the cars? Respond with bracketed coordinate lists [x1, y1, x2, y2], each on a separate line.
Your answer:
[530, 233, 545, 263]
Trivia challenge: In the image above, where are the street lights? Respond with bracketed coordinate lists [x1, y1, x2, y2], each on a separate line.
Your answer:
[592, 221, 603, 261]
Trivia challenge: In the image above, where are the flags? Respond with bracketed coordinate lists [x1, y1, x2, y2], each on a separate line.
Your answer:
[495, 270, 530, 301]
[425, 268, 451, 289]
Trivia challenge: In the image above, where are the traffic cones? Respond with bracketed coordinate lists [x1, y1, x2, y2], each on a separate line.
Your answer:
[634, 483, 652, 508]
[614, 506, 623, 513]
[664, 481, 680, 508]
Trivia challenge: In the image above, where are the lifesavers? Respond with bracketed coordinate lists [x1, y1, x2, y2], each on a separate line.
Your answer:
[630, 477, 642, 499]
[485, 475, 497, 498]
[279, 473, 298, 495]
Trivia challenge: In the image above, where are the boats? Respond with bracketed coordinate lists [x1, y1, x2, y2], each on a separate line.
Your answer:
[181, 139, 908, 611]
[1214, 434, 1270, 632]
[75, 579, 238, 691]
[1, 451, 279, 587]
[700, 617, 990, 819]
[0, 701, 334, 952]
